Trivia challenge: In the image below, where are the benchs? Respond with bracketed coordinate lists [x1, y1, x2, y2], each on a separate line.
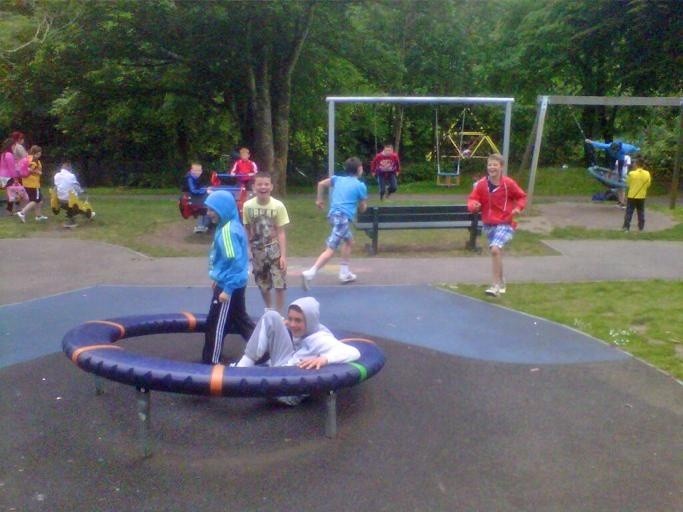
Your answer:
[359, 202, 484, 254]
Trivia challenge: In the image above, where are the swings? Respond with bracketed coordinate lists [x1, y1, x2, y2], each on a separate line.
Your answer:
[571, 104, 640, 188]
[435, 107, 466, 186]
[373, 104, 404, 182]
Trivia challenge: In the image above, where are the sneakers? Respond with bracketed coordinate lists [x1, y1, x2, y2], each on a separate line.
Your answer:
[35, 215, 48, 220]
[302, 272, 311, 291]
[17, 212, 25, 222]
[340, 274, 356, 283]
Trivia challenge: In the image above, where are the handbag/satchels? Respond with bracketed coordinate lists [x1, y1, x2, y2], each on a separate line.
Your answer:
[7, 157, 30, 203]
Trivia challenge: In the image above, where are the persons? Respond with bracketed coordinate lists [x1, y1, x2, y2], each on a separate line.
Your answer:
[226, 295, 363, 408]
[584, 135, 642, 178]
[230, 147, 257, 211]
[240, 173, 291, 313]
[613, 153, 630, 208]
[0, 137, 29, 213]
[197, 189, 269, 374]
[466, 153, 525, 297]
[366, 144, 400, 201]
[180, 161, 218, 234]
[618, 158, 651, 234]
[298, 156, 368, 292]
[15, 144, 49, 224]
[50, 160, 96, 220]
